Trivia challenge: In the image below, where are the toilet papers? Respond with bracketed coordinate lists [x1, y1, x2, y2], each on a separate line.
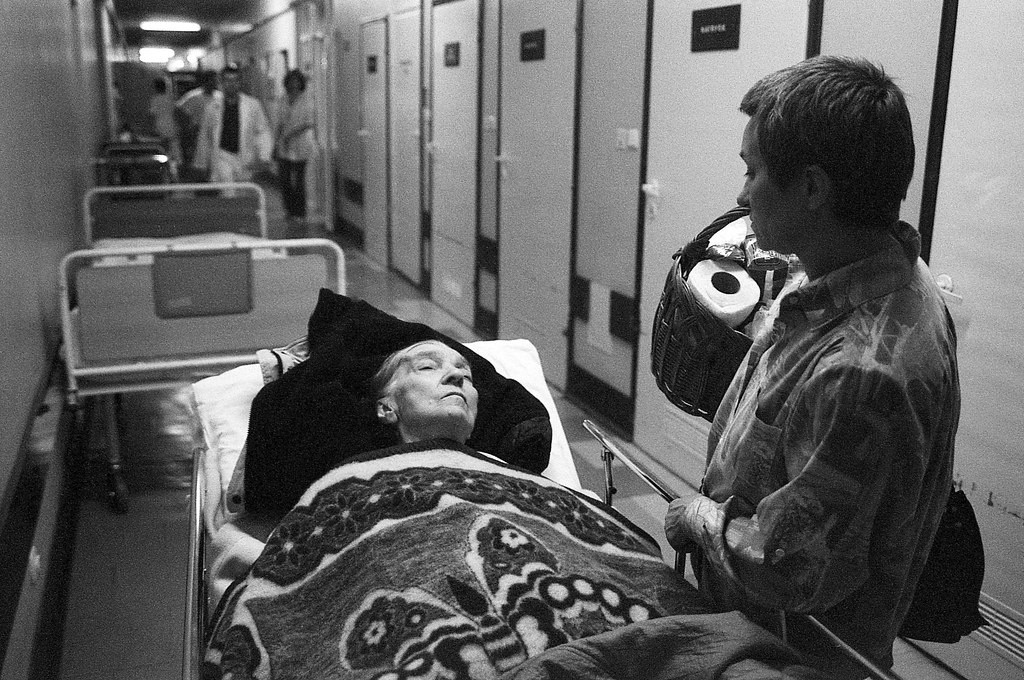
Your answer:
[686, 258, 762, 329]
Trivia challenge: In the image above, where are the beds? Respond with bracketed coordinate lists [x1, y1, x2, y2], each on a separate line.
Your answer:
[177, 340, 897, 680]
[60, 184, 345, 515]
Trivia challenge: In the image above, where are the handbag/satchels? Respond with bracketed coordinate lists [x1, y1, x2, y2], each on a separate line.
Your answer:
[898, 482, 991, 643]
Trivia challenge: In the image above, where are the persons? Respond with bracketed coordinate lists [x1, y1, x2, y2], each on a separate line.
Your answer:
[374, 339, 478, 443]
[274, 69, 317, 221]
[664, 55, 961, 668]
[150, 66, 274, 197]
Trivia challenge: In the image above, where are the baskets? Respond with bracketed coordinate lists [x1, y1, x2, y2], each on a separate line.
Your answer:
[651, 204, 754, 422]
